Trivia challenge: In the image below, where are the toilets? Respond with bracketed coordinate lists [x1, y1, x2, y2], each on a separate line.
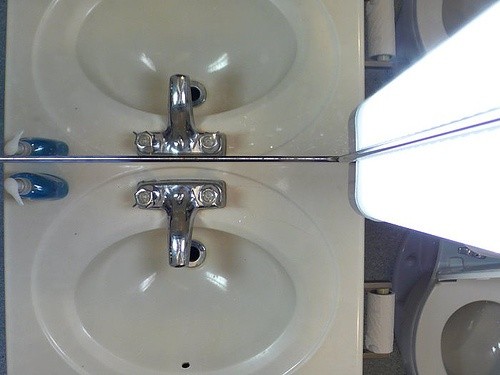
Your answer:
[394, 231, 500, 375]
[364, 0, 494, 90]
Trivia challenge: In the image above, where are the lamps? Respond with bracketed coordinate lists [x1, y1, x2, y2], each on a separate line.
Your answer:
[350, 5, 499, 151]
[347, 116, 499, 258]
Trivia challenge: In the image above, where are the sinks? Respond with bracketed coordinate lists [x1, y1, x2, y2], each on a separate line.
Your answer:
[31, 168, 338, 375]
[32, 0, 342, 153]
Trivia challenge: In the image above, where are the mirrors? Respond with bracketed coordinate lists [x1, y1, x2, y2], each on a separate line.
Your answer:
[0, 0, 498, 161]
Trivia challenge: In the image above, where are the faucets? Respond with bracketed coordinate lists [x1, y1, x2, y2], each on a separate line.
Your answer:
[163, 187, 197, 268]
[167, 74, 194, 133]
